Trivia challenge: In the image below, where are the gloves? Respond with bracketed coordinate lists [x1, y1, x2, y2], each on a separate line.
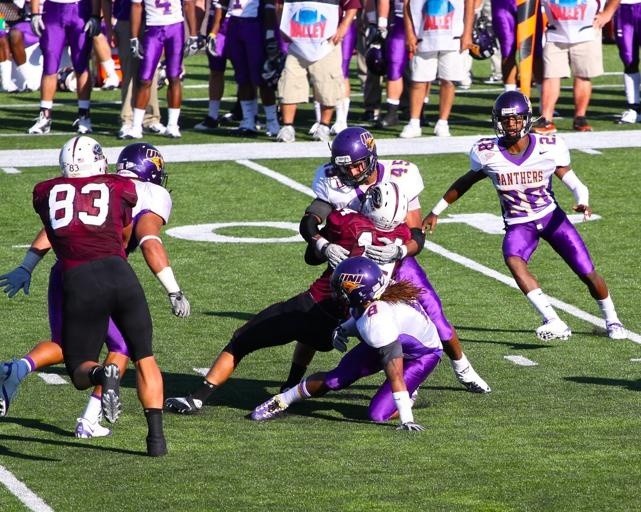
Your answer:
[396, 422, 426, 434]
[1, 266, 31, 298]
[365, 235, 407, 264]
[317, 237, 351, 269]
[330, 326, 350, 353]
[169, 289, 190, 319]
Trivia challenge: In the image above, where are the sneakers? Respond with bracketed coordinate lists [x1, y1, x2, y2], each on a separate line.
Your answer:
[74, 418, 112, 439]
[102, 362, 122, 424]
[27, 115, 51, 134]
[353, 107, 429, 127]
[2, 75, 38, 92]
[0, 358, 15, 418]
[536, 322, 571, 343]
[573, 116, 592, 131]
[398, 119, 422, 138]
[147, 434, 167, 456]
[456, 367, 492, 392]
[434, 120, 450, 136]
[195, 107, 349, 143]
[482, 71, 504, 84]
[251, 387, 294, 421]
[616, 106, 641, 125]
[606, 321, 628, 340]
[101, 74, 120, 90]
[120, 118, 182, 138]
[164, 396, 202, 415]
[78, 112, 92, 134]
[530, 116, 557, 133]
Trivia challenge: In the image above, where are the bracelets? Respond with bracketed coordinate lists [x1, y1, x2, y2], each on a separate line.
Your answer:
[431, 198, 448, 216]
[400, 244, 408, 259]
[169, 290, 185, 300]
[321, 241, 332, 258]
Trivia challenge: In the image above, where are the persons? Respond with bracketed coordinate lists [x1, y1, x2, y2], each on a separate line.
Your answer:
[281, 127, 492, 395]
[32, 136, 166, 456]
[253, 255, 442, 431]
[421, 91, 628, 341]
[1, 144, 190, 437]
[165, 182, 412, 416]
[1, 0, 641, 140]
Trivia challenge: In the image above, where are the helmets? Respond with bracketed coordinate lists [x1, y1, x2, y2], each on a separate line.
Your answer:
[116, 142, 168, 187]
[330, 255, 384, 309]
[362, 23, 390, 75]
[361, 182, 409, 232]
[56, 65, 77, 91]
[492, 91, 532, 144]
[331, 127, 376, 186]
[60, 135, 108, 179]
[470, 16, 499, 60]
